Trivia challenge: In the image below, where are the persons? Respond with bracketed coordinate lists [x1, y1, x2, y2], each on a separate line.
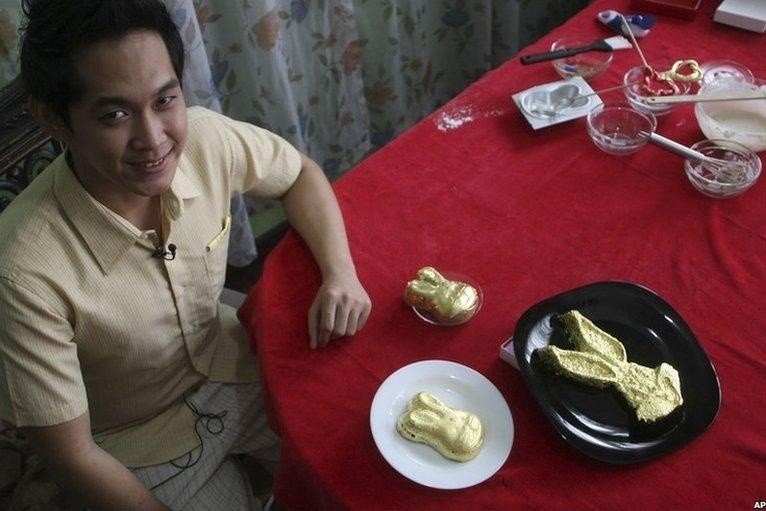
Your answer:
[0, 1, 373, 510]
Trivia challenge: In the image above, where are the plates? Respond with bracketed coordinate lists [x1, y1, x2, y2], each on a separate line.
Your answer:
[412, 273, 482, 327]
[515, 281, 724, 468]
[368, 360, 515, 491]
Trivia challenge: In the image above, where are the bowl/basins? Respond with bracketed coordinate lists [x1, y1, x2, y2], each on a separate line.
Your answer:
[551, 35, 766, 206]
